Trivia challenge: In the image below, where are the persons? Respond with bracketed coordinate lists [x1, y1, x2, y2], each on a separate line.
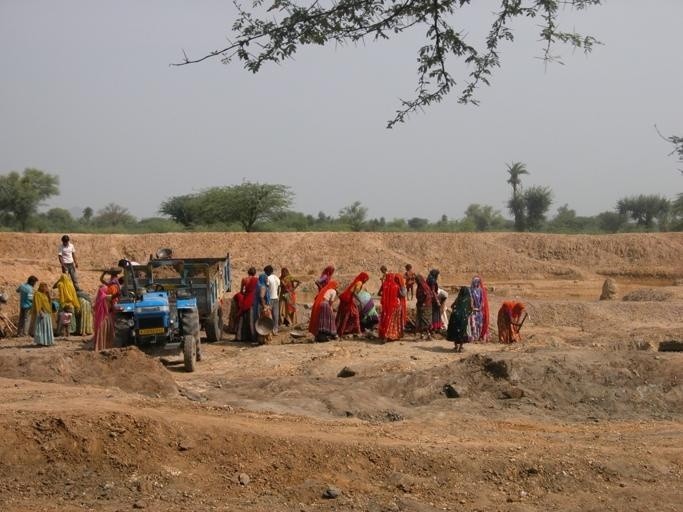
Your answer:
[496, 300, 526, 345]
[227, 263, 490, 354]
[57, 234, 81, 292]
[0, 268, 122, 352]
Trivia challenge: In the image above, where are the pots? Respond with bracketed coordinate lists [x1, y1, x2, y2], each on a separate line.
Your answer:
[291, 331, 308, 339]
[255, 316, 274, 337]
[157, 249, 174, 259]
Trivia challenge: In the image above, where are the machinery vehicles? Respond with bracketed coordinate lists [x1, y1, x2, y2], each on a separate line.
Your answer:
[108, 248, 233, 371]
